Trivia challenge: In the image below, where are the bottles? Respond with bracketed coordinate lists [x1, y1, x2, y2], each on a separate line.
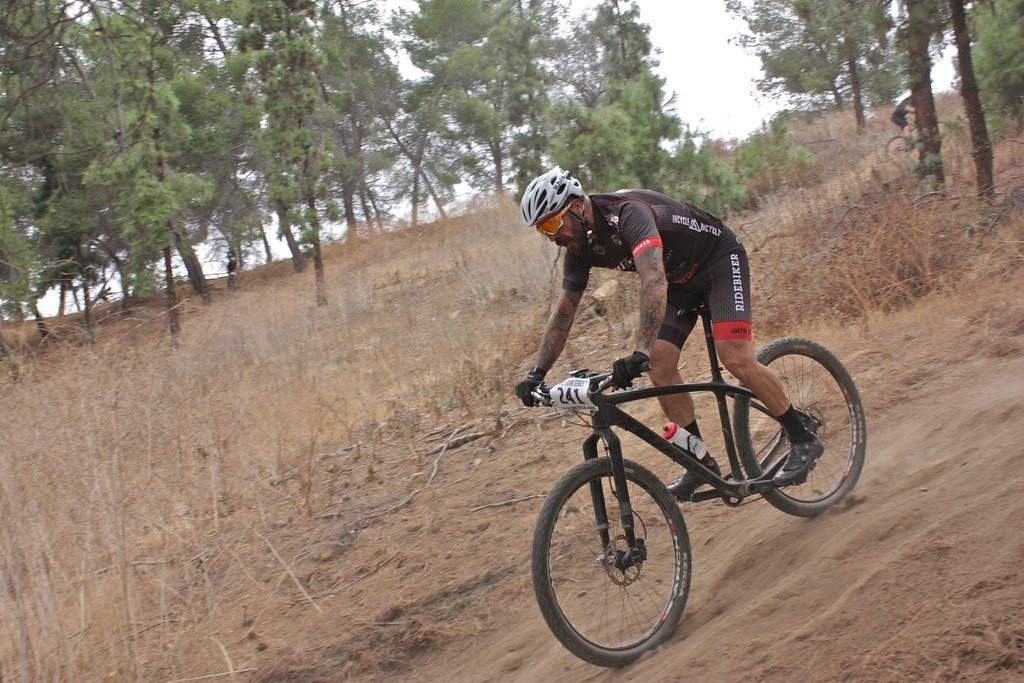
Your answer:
[662, 422, 707, 459]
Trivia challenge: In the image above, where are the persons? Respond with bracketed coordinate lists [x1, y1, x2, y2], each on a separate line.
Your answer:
[892, 91, 916, 138]
[511, 166, 825, 499]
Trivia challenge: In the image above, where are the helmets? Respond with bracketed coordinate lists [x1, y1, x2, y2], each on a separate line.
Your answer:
[520, 164, 585, 227]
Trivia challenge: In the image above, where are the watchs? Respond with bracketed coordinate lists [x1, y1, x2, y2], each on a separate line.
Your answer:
[528, 366, 546, 378]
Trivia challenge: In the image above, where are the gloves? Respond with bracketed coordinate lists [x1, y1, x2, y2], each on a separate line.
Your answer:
[613, 351, 650, 391]
[515, 373, 543, 407]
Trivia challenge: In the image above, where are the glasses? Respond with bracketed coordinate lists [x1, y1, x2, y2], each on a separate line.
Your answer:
[536, 201, 574, 235]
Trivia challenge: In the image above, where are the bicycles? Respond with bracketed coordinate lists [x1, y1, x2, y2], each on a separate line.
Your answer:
[531, 284, 867, 667]
[883, 122, 950, 167]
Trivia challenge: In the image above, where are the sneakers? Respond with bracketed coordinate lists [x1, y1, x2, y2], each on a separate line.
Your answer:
[772, 432, 824, 487]
[666, 457, 721, 502]
